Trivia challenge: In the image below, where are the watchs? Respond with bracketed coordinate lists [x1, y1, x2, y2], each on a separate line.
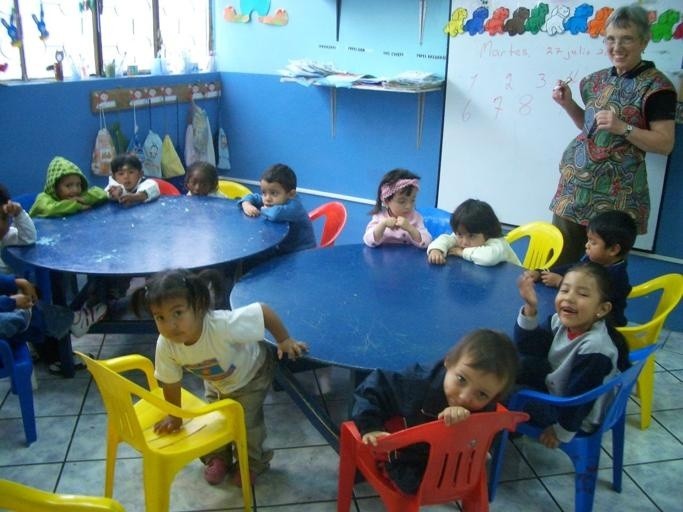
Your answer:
[623, 124, 633, 138]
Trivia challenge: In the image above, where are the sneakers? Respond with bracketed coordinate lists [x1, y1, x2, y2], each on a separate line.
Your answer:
[69, 297, 108, 342]
[231, 467, 256, 487]
[202, 455, 228, 485]
[46, 349, 96, 376]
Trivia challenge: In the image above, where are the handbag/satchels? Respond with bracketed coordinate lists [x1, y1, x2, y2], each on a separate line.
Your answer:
[184, 106, 216, 169]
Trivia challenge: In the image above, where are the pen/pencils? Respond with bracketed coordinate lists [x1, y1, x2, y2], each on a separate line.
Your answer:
[553, 78, 571, 92]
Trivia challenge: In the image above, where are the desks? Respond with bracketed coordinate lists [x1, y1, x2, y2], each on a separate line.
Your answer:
[3, 194, 292, 383]
[229, 244, 568, 484]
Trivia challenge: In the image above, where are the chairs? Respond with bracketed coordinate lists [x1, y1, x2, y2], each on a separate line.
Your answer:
[1, 337, 41, 452]
[145, 175, 181, 197]
[606, 267, 682, 429]
[482, 340, 660, 511]
[66, 344, 259, 511]
[303, 199, 349, 248]
[1, 475, 122, 511]
[329, 403, 531, 512]
[499, 222, 566, 277]
[216, 179, 253, 203]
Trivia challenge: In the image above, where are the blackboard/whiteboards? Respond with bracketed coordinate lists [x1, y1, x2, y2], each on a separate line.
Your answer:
[435, 1, 683, 253]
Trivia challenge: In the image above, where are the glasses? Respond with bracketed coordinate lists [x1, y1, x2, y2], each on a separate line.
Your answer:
[602, 32, 644, 48]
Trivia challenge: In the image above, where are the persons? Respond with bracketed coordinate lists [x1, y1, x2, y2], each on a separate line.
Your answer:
[344, 328, 521, 492]
[426, 200, 522, 266]
[537, 2, 677, 269]
[523, 210, 639, 334]
[508, 261, 627, 451]
[130, 269, 310, 489]
[363, 169, 433, 250]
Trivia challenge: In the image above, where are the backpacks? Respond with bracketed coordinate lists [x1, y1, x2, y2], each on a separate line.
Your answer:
[90, 126, 116, 176]
[126, 130, 144, 170]
[214, 127, 232, 172]
[161, 134, 186, 181]
[140, 128, 164, 179]
